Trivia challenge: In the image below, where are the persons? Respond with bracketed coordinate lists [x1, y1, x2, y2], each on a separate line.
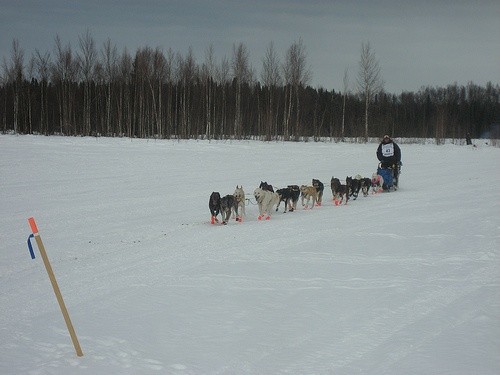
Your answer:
[377, 135, 400, 180]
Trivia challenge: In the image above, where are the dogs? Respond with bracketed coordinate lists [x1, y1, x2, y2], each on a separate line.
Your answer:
[233, 184, 246, 223]
[312, 178, 325, 206]
[300, 185, 321, 210]
[276, 187, 296, 214]
[259, 181, 275, 193]
[287, 184, 300, 210]
[220, 195, 239, 225]
[329, 176, 349, 206]
[254, 186, 280, 221]
[345, 175, 362, 200]
[371, 171, 384, 194]
[360, 177, 375, 197]
[354, 174, 365, 180]
[208, 190, 221, 225]
